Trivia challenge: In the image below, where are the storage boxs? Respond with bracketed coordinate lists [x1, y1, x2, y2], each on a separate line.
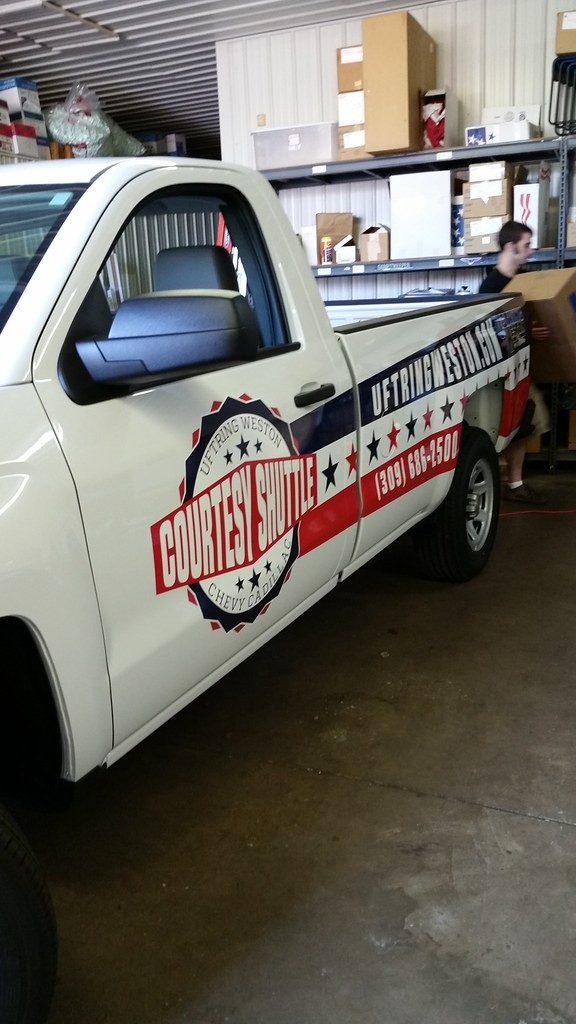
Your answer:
[250, 10, 459, 172]
[333, 234, 356, 264]
[554, 10, 576, 55]
[388, 160, 552, 259]
[559, 405, 576, 450]
[136, 132, 187, 158]
[500, 268, 576, 386]
[359, 223, 389, 262]
[0, 78, 52, 165]
[565, 206, 576, 247]
[465, 119, 539, 147]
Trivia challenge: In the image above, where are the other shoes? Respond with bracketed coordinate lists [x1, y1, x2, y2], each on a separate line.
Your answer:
[503, 484, 546, 503]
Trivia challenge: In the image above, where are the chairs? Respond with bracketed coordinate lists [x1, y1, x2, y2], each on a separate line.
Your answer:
[151, 245, 239, 293]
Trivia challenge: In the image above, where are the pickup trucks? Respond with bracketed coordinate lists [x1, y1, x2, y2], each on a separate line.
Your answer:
[0, 153, 536, 1024]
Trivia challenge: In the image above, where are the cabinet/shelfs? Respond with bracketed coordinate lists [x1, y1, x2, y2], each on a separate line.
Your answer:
[258, 136, 576, 477]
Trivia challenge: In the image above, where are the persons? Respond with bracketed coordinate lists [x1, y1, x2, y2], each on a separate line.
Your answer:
[479, 223, 551, 501]
